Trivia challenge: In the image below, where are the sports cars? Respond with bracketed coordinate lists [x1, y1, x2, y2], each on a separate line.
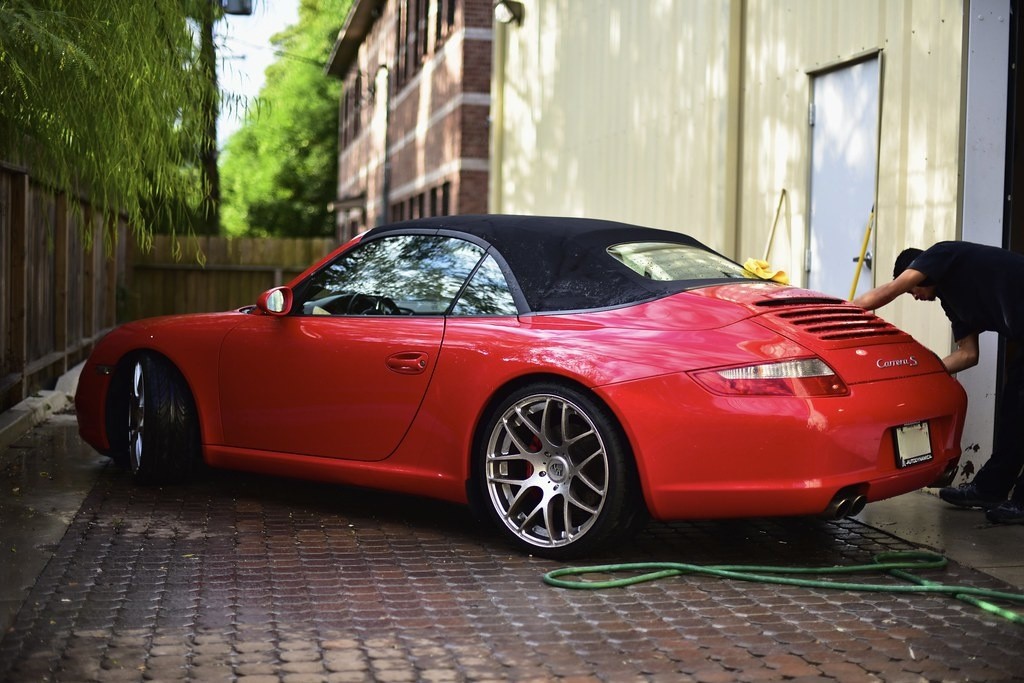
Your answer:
[74, 214, 968, 561]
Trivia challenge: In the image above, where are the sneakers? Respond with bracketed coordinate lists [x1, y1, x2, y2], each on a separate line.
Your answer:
[939, 482, 997, 508]
[986, 500, 1024, 524]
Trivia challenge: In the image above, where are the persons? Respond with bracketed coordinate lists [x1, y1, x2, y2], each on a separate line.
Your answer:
[852, 241, 1024, 523]
